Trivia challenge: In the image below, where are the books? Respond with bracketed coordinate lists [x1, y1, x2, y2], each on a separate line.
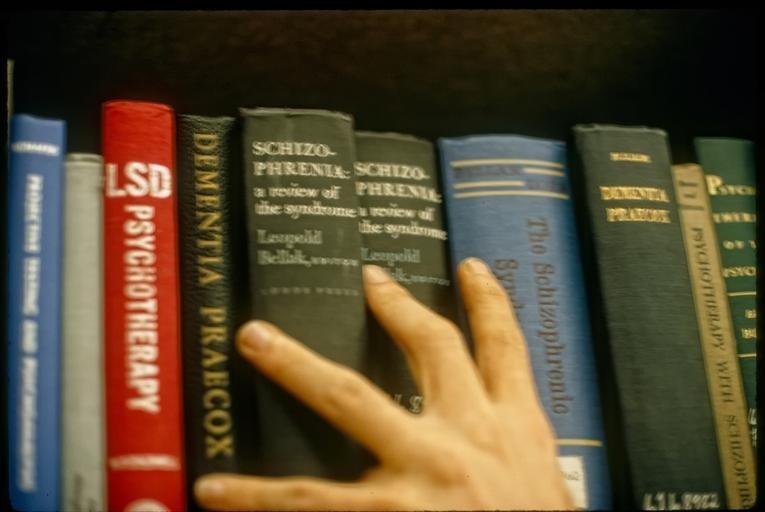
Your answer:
[3, 96, 765, 511]
